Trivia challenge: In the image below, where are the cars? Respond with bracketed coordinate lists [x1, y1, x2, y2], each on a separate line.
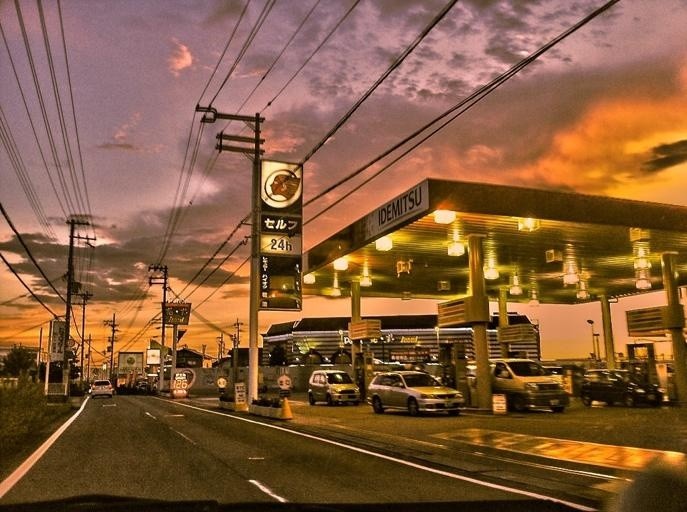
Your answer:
[367, 370, 465, 417]
[91, 380, 113, 399]
[580, 368, 664, 407]
[309, 369, 361, 405]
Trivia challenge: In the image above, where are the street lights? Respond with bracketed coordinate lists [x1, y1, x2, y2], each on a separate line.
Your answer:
[587, 319, 600, 360]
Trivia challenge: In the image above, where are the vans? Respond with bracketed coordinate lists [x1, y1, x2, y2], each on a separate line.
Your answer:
[468, 358, 569, 413]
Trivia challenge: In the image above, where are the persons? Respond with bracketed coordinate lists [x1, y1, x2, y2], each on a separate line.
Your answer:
[441, 365, 456, 389]
